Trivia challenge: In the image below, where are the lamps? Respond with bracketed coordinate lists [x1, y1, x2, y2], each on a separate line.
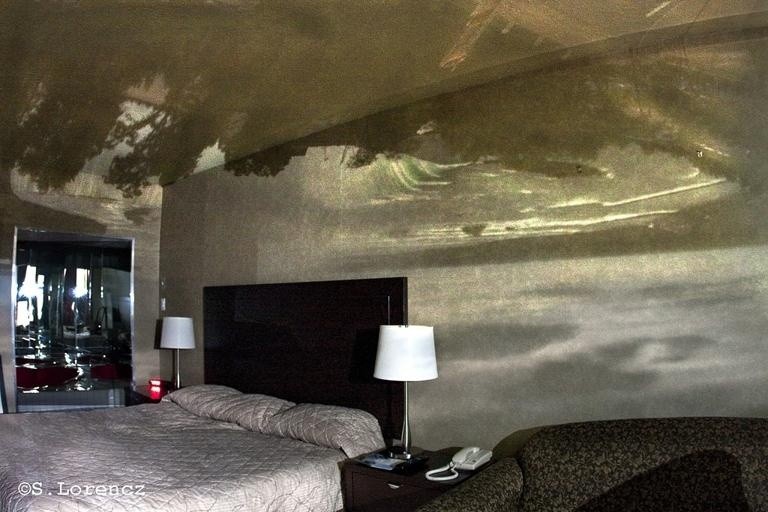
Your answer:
[160, 316, 196, 394]
[373, 324, 438, 477]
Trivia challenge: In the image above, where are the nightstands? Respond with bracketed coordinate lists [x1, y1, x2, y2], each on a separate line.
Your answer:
[125, 383, 185, 406]
[338, 445, 498, 512]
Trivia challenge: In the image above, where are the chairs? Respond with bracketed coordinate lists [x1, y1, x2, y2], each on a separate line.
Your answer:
[411, 417, 768, 512]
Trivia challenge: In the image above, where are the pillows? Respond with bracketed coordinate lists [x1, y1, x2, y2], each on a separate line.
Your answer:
[161, 384, 385, 457]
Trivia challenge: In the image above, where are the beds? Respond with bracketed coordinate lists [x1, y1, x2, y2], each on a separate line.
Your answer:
[0, 276, 407, 512]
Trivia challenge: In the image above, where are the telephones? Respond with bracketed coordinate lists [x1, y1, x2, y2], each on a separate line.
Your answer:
[450, 447, 492, 470]
